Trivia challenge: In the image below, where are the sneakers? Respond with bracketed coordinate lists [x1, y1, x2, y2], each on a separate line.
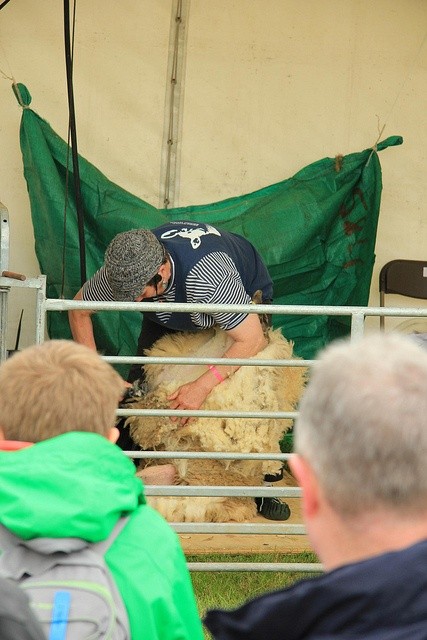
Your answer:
[255, 493, 291, 521]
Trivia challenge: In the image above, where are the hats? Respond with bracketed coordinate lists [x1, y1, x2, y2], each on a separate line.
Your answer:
[104, 229, 166, 301]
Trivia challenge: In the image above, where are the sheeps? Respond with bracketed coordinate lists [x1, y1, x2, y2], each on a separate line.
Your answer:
[119, 290, 307, 524]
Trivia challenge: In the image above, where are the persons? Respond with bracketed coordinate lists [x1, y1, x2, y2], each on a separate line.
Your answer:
[1, 339, 202, 640]
[66, 219, 272, 464]
[202, 331, 427, 640]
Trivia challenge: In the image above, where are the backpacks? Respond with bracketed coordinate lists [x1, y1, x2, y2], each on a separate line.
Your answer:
[1, 513, 130, 640]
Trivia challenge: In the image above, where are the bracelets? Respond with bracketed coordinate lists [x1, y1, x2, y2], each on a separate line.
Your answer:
[208, 363, 223, 383]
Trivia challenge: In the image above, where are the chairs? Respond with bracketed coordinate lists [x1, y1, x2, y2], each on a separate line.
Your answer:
[375, 259, 427, 339]
